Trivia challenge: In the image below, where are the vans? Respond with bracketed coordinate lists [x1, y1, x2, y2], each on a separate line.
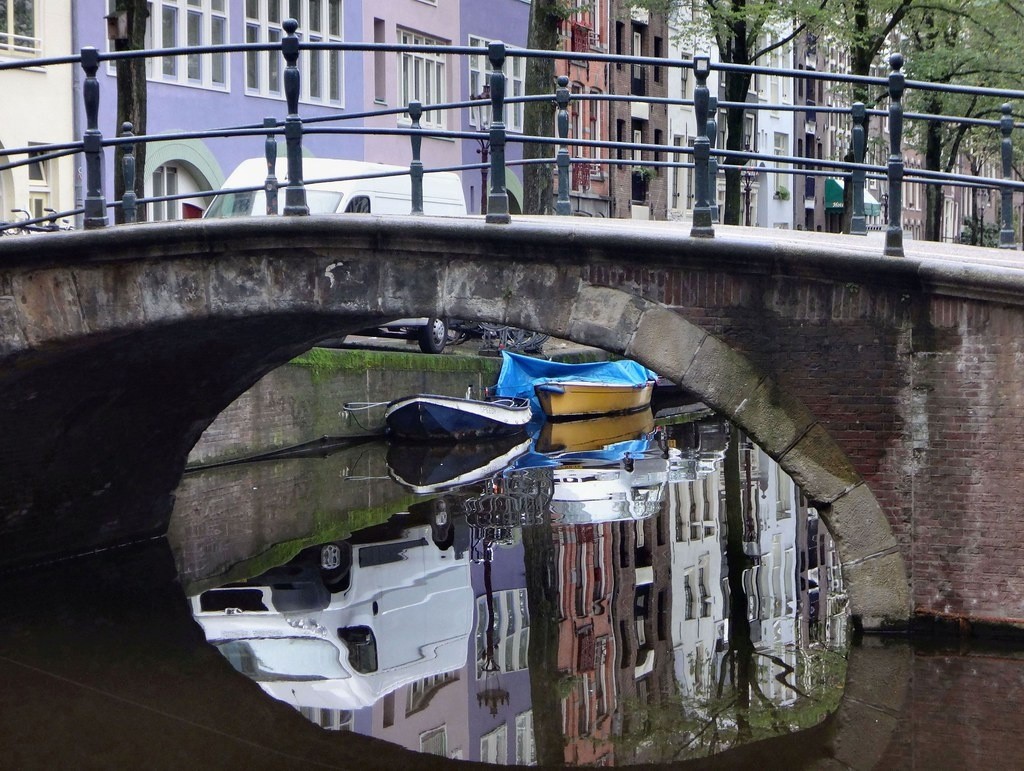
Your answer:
[201, 501, 478, 710]
[198, 156, 466, 353]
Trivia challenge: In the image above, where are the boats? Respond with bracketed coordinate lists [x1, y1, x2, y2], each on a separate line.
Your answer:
[384, 437, 535, 497]
[536, 407, 658, 454]
[384, 391, 533, 439]
[534, 365, 656, 419]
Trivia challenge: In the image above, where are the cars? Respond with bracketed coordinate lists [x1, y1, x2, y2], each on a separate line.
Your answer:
[548, 425, 670, 528]
